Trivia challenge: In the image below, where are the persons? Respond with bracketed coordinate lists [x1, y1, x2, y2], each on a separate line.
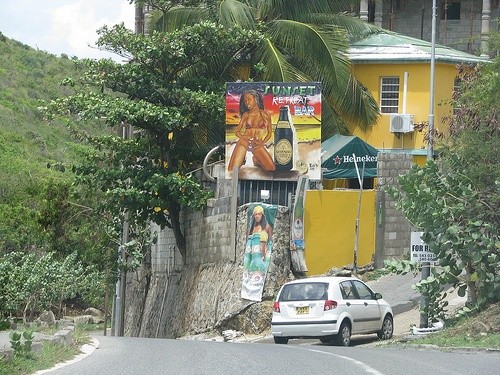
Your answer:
[245, 206, 272, 272]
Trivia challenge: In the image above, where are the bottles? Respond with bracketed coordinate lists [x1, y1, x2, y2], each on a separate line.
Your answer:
[273, 107, 294, 171]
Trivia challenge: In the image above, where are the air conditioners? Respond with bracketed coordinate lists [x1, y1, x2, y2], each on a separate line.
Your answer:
[390, 112, 414, 134]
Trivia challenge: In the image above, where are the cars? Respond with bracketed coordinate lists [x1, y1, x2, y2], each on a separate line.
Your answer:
[271, 276, 394, 347]
